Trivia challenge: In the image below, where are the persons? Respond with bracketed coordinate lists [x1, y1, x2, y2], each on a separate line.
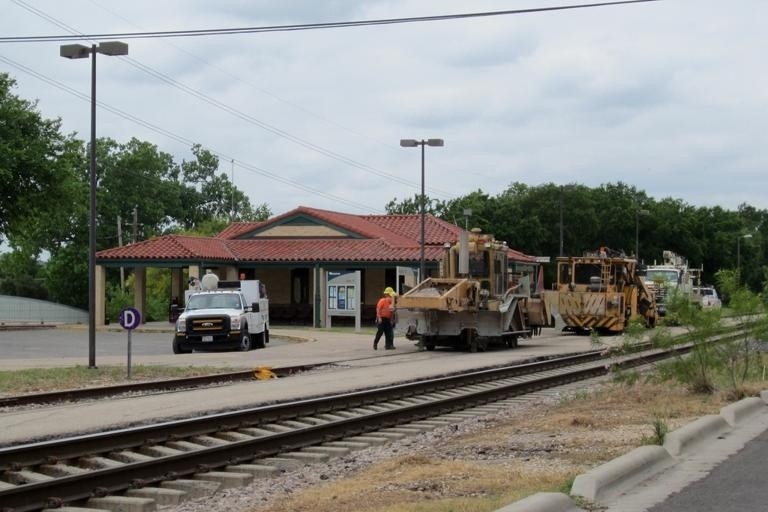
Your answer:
[372, 287, 397, 351]
[205, 269, 212, 274]
[518, 270, 531, 297]
[238, 271, 246, 280]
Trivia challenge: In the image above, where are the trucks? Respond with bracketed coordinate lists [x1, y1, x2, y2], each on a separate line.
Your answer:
[166, 279, 272, 354]
[637, 248, 704, 318]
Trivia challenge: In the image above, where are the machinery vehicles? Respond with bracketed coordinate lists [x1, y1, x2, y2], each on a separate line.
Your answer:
[390, 225, 557, 353]
[550, 246, 663, 336]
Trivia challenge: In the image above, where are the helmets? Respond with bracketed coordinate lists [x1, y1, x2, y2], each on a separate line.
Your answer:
[383, 287, 392, 294]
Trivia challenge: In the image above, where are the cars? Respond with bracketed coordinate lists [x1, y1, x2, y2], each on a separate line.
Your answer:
[691, 286, 723, 310]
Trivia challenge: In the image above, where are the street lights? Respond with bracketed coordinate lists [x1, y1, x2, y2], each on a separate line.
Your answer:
[634, 209, 650, 260]
[737, 234, 754, 268]
[57, 39, 132, 371]
[399, 138, 445, 282]
